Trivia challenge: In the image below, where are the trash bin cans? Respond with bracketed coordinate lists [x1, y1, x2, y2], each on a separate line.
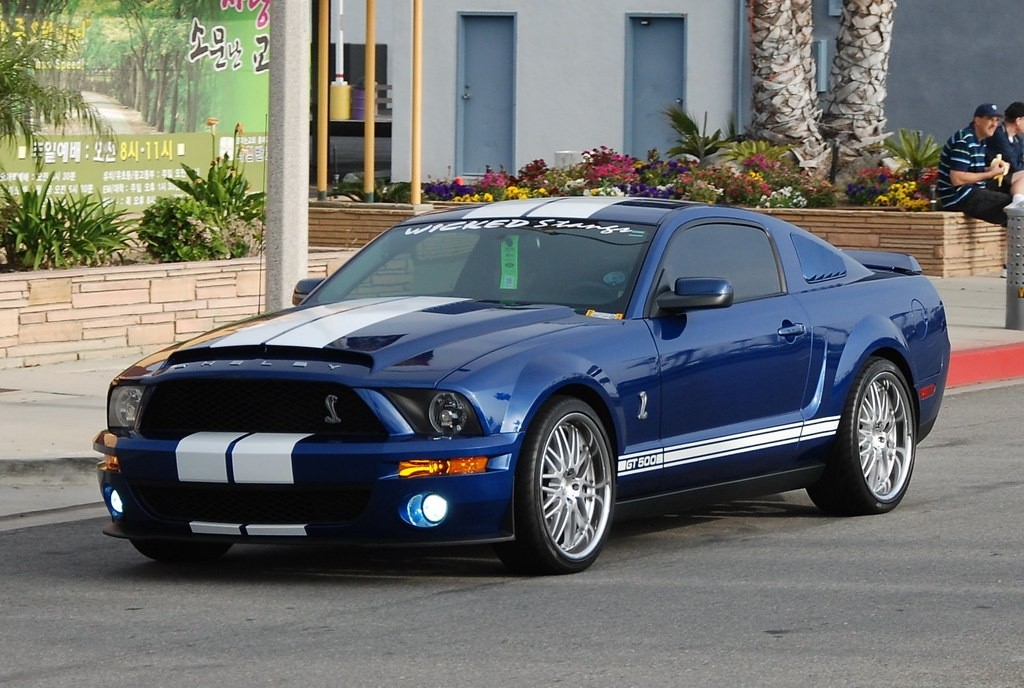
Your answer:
[1003, 209, 1024, 331]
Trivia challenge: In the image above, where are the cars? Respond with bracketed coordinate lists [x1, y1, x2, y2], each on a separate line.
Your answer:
[92, 196, 949, 581]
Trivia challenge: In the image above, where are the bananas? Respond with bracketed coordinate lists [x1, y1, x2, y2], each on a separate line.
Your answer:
[990, 153, 1010, 187]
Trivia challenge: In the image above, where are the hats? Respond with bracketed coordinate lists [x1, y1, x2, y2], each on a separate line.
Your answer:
[974, 103, 1005, 118]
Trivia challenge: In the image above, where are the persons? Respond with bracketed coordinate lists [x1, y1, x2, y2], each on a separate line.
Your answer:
[937, 101, 1024, 228]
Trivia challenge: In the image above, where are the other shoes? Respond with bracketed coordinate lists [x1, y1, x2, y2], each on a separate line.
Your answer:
[1001, 268, 1007, 278]
[1003, 203, 1023, 212]
